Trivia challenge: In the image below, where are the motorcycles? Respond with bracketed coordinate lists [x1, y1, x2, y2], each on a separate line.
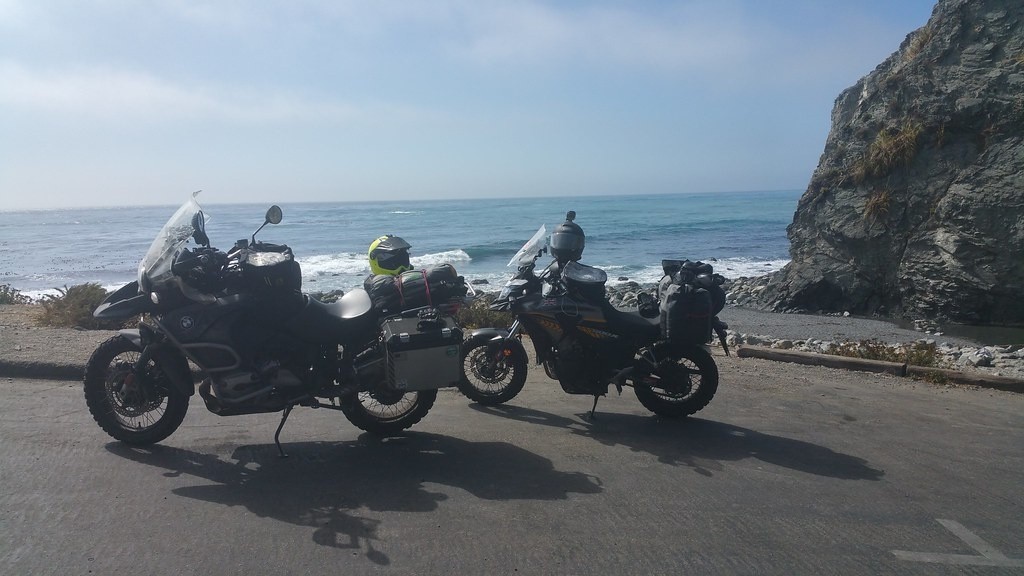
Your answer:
[83, 199, 490, 461]
[455, 211, 731, 424]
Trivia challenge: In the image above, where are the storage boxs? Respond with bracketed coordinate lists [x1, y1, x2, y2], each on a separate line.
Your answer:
[381, 314, 464, 390]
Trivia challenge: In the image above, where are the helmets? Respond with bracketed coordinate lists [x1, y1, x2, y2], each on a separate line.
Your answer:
[369, 234, 414, 275]
[550, 211, 585, 261]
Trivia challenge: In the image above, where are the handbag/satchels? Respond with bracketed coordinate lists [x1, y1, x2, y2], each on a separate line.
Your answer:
[560, 260, 608, 294]
